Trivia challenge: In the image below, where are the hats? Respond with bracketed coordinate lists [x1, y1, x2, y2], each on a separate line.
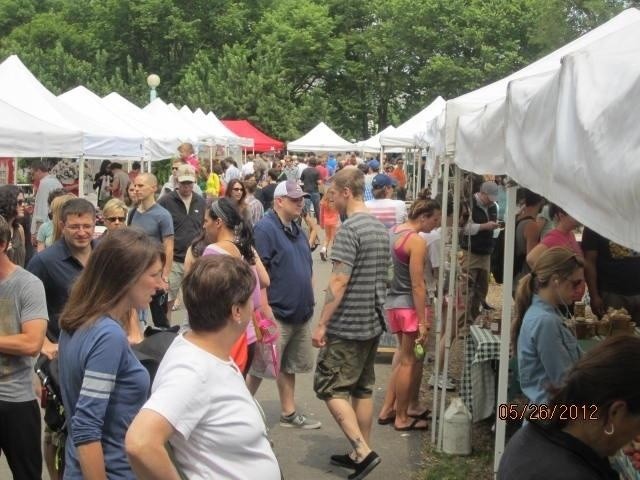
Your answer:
[365, 159, 379, 169]
[481, 181, 500, 201]
[177, 164, 195, 182]
[232, 188, 244, 191]
[274, 180, 313, 201]
[372, 174, 397, 188]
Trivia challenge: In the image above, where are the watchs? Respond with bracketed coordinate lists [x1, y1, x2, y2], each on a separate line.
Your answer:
[162, 277, 169, 283]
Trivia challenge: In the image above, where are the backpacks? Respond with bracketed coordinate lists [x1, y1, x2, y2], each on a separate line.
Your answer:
[490, 215, 536, 284]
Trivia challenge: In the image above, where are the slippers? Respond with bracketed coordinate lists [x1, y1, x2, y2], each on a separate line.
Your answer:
[407, 409, 439, 419]
[394, 418, 428, 430]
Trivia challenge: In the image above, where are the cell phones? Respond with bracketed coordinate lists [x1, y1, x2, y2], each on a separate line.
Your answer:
[497, 221, 505, 225]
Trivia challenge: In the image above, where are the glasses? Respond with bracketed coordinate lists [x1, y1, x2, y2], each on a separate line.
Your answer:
[17, 199, 26, 204]
[565, 277, 582, 289]
[105, 217, 126, 222]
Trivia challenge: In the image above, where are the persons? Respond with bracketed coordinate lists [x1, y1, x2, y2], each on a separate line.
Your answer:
[300, 158, 321, 225]
[319, 189, 339, 261]
[262, 168, 279, 211]
[199, 198, 259, 382]
[392, 159, 406, 200]
[242, 154, 255, 176]
[156, 160, 203, 311]
[94, 199, 128, 244]
[36, 189, 69, 253]
[300, 198, 317, 251]
[428, 300, 458, 390]
[207, 165, 223, 197]
[184, 234, 270, 289]
[358, 163, 374, 201]
[316, 160, 328, 192]
[365, 173, 407, 229]
[367, 160, 379, 177]
[379, 198, 442, 431]
[297, 157, 307, 191]
[25, 198, 97, 480]
[326, 152, 337, 175]
[392, 340, 435, 419]
[93, 160, 112, 207]
[278, 156, 301, 183]
[512, 190, 546, 301]
[164, 143, 200, 191]
[235, 179, 264, 242]
[124, 179, 137, 225]
[244, 174, 265, 205]
[1, 217, 48, 480]
[496, 333, 640, 480]
[311, 165, 393, 480]
[510, 245, 585, 426]
[536, 205, 553, 240]
[0, 184, 26, 268]
[30, 159, 64, 245]
[57, 226, 168, 479]
[123, 254, 282, 480]
[459, 181, 502, 319]
[420, 192, 461, 298]
[581, 226, 640, 326]
[222, 156, 240, 184]
[225, 179, 246, 203]
[526, 201, 586, 316]
[106, 163, 129, 201]
[156, 162, 207, 323]
[126, 171, 174, 329]
[126, 161, 141, 203]
[272, 160, 288, 183]
[246, 179, 322, 429]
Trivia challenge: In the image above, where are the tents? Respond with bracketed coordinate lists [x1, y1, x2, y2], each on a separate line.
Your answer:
[208, 110, 254, 172]
[414, 7, 639, 441]
[359, 124, 419, 163]
[0, 52, 85, 199]
[287, 122, 361, 156]
[380, 96, 445, 172]
[220, 119, 284, 159]
[102, 92, 213, 172]
[435, 4, 640, 478]
[56, 84, 144, 173]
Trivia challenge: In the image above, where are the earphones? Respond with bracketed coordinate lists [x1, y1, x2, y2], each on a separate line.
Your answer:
[555, 279, 559, 286]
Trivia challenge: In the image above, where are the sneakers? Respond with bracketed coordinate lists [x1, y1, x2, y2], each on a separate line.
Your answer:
[320, 247, 327, 262]
[330, 454, 360, 469]
[348, 451, 381, 480]
[280, 411, 322, 429]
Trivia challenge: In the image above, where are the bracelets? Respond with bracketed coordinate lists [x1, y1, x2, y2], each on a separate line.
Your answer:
[418, 324, 425, 327]
